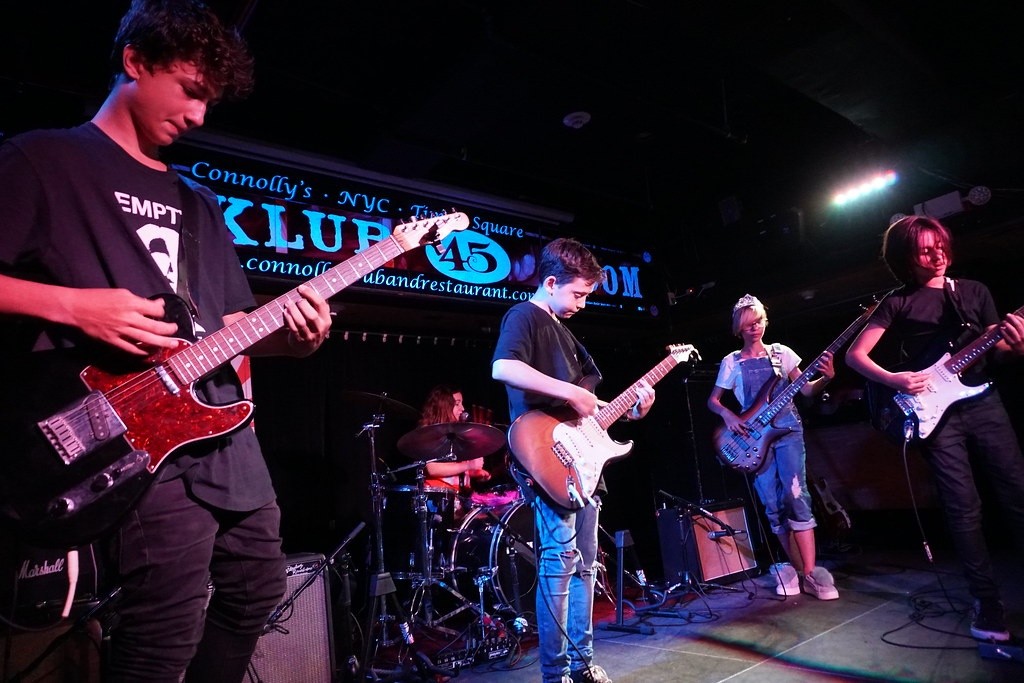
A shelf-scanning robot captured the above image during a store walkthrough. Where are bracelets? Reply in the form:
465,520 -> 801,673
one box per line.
821,377 -> 829,385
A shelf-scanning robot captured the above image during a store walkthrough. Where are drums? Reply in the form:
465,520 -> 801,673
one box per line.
449,501 -> 541,636
370,485 -> 456,581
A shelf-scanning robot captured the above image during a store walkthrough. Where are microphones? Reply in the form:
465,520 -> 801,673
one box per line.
707,530 -> 747,539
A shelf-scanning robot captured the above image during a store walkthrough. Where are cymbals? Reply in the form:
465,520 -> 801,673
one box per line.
397,422 -> 507,463
343,391 -> 422,421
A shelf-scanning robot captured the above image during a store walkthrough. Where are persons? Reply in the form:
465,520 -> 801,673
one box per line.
0,0 -> 332,682
413,388 -> 491,521
708,293 -> 839,600
843,215 -> 1024,643
492,239 -> 658,683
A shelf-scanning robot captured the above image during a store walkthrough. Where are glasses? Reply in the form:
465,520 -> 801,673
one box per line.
742,319 -> 766,331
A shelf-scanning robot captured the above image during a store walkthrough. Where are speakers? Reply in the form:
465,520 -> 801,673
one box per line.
178,553 -> 337,683
656,497 -> 763,588
8,543 -> 99,612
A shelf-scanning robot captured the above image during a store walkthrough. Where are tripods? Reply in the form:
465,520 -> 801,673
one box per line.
657,489 -> 743,609
355,400 -> 539,683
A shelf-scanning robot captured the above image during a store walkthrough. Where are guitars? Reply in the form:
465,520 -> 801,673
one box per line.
0,207 -> 477,550
710,293 -> 885,476
506,343 -> 695,514
864,306 -> 1024,451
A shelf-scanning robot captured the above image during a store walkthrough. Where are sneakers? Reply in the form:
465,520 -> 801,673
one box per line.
971,600 -> 1009,641
803,567 -> 840,600
569,664 -> 613,683
559,675 -> 574,683
776,566 -> 802,595
